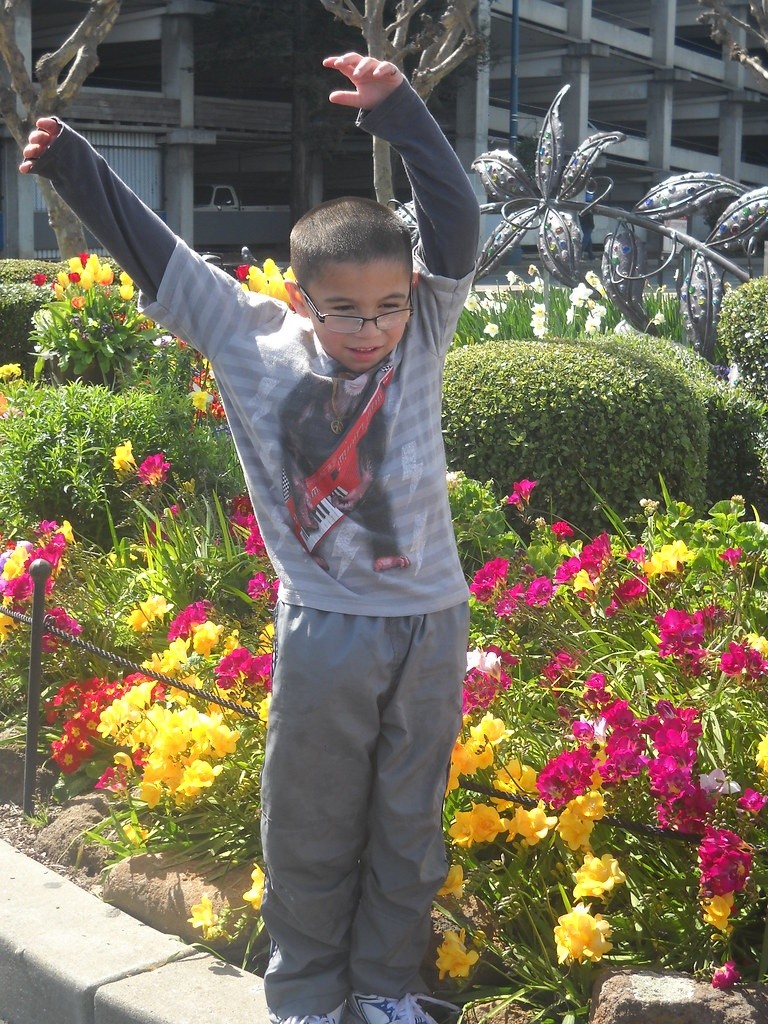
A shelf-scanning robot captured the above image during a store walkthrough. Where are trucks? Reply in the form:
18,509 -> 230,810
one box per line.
192,184 -> 291,213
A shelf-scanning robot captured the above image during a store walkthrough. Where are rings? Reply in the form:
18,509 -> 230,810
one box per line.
391,66 -> 397,76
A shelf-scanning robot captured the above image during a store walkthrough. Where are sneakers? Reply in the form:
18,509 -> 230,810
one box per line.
267,998 -> 346,1024
345,992 -> 458,1024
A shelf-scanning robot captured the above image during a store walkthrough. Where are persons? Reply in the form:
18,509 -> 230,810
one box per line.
17,52 -> 479,1024
579,196 -> 595,259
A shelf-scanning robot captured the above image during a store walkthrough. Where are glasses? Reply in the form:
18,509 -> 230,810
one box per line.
296,277 -> 415,334
